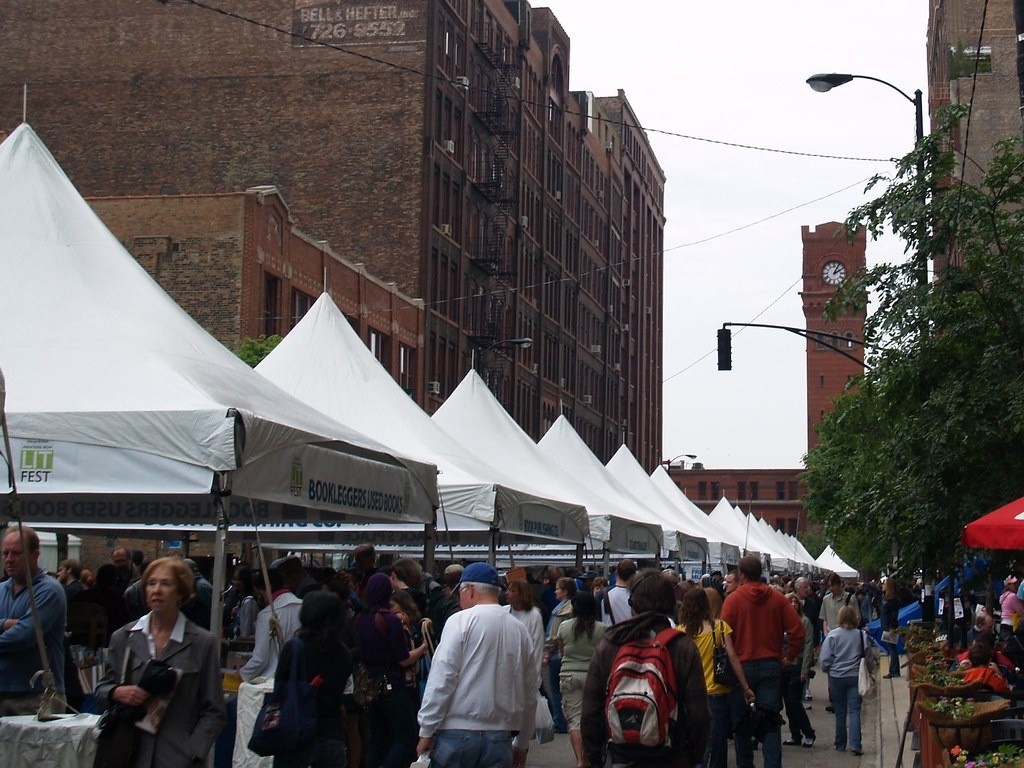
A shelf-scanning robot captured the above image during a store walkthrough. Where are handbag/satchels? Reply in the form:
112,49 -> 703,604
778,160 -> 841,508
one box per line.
93,703 -> 147,768
247,639 -> 323,758
353,665 -> 393,713
712,619 -> 737,685
536,691 -> 555,744
858,631 -> 878,698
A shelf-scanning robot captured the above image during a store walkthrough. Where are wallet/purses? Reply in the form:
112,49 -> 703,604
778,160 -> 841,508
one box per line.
130,660 -> 183,734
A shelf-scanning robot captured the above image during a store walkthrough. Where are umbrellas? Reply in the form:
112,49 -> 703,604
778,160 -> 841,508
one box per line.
961,497 -> 1024,549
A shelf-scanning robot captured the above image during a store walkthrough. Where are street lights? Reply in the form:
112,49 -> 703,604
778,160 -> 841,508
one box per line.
806,72 -> 932,348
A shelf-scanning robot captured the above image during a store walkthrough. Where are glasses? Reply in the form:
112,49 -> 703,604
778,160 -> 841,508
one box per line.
453,584 -> 473,602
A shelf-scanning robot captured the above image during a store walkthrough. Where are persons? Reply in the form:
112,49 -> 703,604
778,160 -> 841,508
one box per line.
960,574 -> 1024,696
0,528 -> 921,768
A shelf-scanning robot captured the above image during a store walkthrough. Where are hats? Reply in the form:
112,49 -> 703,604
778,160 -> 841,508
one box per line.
577,571 -> 597,579
1003,575 -> 1020,585
451,562 -> 499,594
354,546 -> 374,562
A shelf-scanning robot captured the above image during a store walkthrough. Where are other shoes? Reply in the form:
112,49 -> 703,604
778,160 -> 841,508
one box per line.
803,737 -> 815,747
783,741 -> 801,745
850,747 -> 864,755
883,674 -> 899,678
802,703 -> 812,709
825,707 -> 835,713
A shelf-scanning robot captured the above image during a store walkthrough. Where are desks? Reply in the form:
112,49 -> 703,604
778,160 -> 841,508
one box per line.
0,711 -> 103,768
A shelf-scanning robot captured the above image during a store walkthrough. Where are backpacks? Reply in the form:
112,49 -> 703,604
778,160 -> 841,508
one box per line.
604,628 -> 687,768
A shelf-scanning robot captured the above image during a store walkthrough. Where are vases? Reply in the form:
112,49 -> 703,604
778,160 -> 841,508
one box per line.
903,631 -> 1011,768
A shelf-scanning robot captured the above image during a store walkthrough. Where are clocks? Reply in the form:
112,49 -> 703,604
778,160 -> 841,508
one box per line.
821,261 -> 850,287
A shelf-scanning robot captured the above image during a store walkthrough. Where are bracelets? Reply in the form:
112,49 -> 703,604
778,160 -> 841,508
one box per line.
745,687 -> 749,691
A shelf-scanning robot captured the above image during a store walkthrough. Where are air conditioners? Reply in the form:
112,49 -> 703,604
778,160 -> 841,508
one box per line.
610,305 -> 613,312
594,239 -> 599,248
512,76 -> 520,89
442,224 -> 452,235
624,278 -> 633,287
615,362 -> 621,371
521,216 -> 531,227
456,76 -> 470,89
532,364 -> 538,372
620,419 -> 627,425
429,381 -> 440,393
623,323 -> 630,330
647,307 -> 652,313
561,378 -> 566,388
606,141 -> 617,151
597,191 -> 604,198
592,345 -> 602,355
443,140 -> 456,154
583,395 -> 592,404
556,191 -> 562,200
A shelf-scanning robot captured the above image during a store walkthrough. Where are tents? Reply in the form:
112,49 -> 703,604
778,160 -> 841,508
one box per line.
0,123 -> 860,659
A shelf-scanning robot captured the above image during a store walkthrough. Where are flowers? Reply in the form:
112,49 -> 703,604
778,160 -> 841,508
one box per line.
898,623 -> 938,641
914,672 -> 972,687
909,635 -> 948,648
925,655 -> 971,674
920,650 -> 946,666
951,743 -> 1024,768
928,695 -> 985,720
